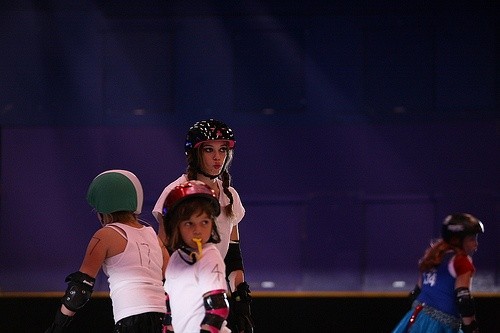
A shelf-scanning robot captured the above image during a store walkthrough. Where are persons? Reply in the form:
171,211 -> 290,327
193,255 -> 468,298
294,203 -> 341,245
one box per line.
151,117 -> 255,333
162,182 -> 230,333
393,213 -> 485,333
54,170 -> 170,333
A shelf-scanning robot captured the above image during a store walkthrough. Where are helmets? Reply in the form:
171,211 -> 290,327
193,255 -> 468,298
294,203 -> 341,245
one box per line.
440,212 -> 485,245
182,119 -> 237,152
86,169 -> 144,215
160,179 -> 221,218
